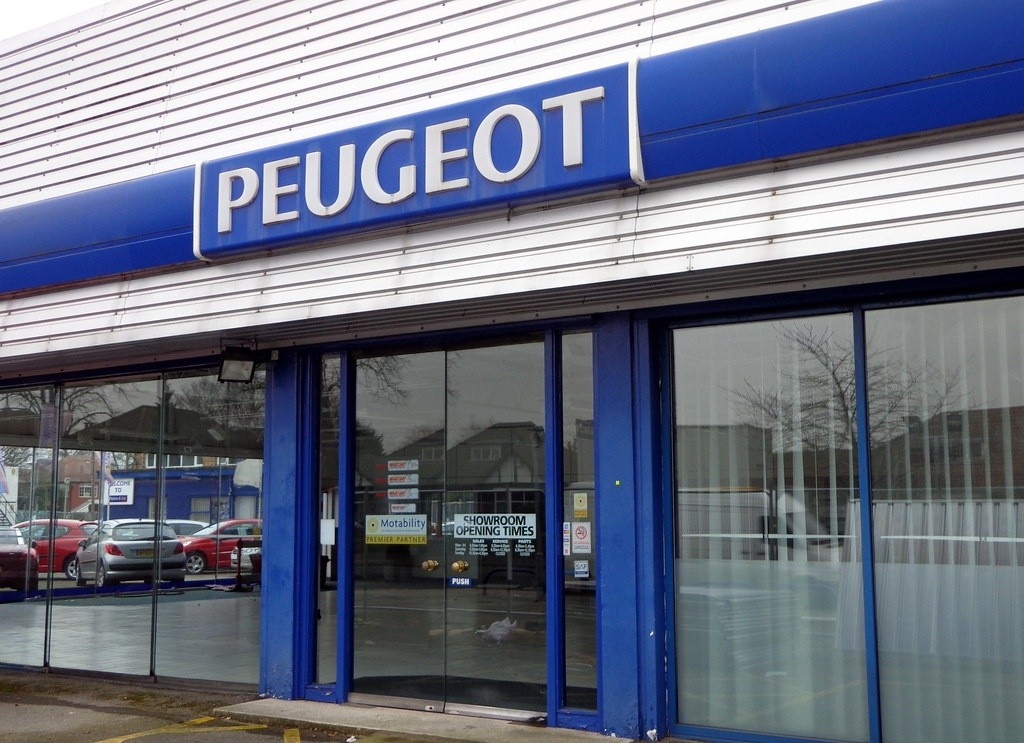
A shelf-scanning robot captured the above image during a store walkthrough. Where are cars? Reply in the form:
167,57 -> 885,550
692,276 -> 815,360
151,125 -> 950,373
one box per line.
75,517 -> 187,587
0,526 -> 39,591
178,517 -> 263,576
160,519 -> 210,539
10,518 -> 98,584
229,547 -> 261,572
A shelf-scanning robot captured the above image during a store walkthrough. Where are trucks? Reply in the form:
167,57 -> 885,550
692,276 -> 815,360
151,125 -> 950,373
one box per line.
675,482 -> 840,641
